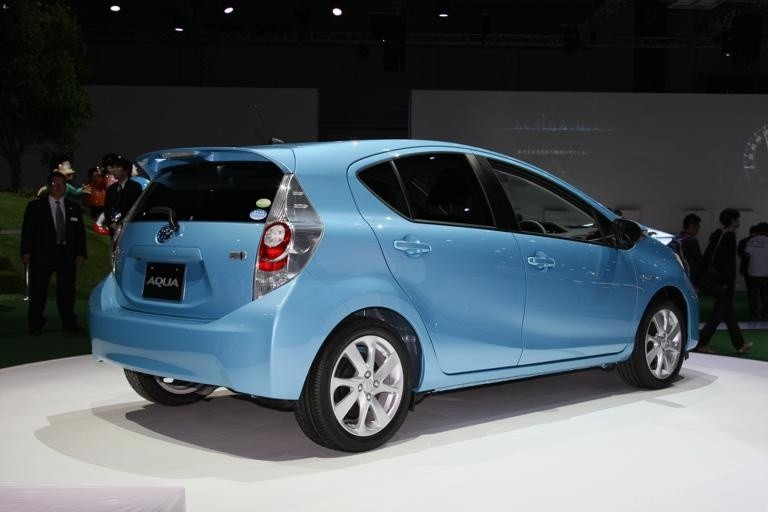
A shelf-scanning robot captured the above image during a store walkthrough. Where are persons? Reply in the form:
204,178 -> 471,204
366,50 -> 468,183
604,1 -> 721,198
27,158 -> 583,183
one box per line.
677,213 -> 701,294
694,208 -> 754,354
743,223 -> 768,321
21,153 -> 142,335
737,225 -> 757,317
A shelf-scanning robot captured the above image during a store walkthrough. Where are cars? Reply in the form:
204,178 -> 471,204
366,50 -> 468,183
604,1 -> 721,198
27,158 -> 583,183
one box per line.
87,139 -> 701,452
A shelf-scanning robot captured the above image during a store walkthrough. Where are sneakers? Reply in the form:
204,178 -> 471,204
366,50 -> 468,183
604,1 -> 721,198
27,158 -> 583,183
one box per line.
736,342 -> 753,354
697,342 -> 715,353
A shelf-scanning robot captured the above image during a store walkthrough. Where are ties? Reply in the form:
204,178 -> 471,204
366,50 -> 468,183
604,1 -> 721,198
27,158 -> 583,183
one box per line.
55,201 -> 64,243
117,184 -> 123,190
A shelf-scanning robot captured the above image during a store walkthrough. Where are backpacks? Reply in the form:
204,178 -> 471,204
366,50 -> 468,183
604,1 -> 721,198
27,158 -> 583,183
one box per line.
667,235 -> 691,277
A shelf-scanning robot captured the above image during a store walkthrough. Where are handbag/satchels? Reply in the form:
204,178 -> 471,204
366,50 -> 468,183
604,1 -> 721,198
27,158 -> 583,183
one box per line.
698,262 -> 724,297
